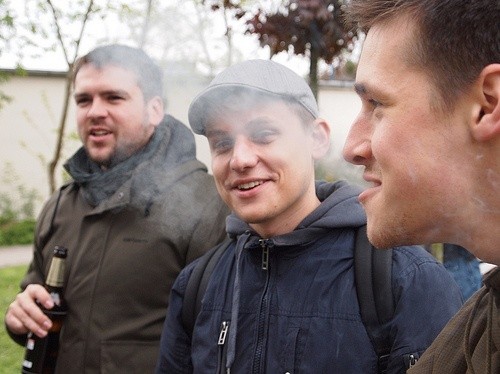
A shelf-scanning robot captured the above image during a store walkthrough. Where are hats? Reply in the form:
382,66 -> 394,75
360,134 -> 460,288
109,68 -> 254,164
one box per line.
188,59 -> 318,136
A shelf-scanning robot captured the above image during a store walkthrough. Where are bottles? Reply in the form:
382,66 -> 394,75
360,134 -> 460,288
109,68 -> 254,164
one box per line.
22,246 -> 68,374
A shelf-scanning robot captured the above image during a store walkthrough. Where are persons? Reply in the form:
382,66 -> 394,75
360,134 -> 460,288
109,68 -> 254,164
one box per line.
10,48 -> 232,374
341,0 -> 499,374
153,59 -> 465,374
